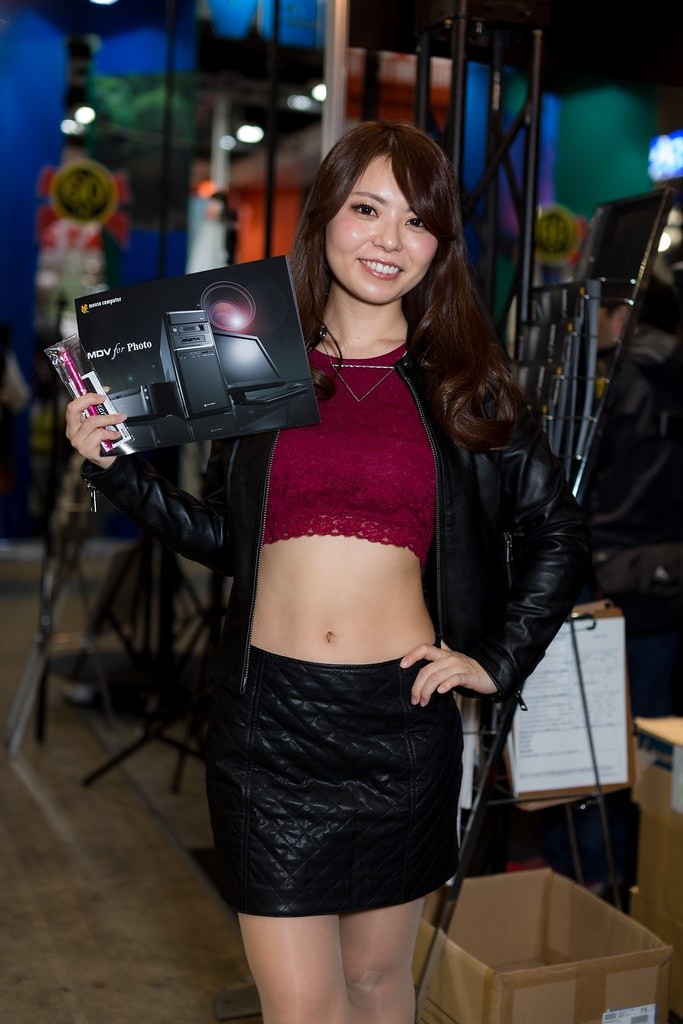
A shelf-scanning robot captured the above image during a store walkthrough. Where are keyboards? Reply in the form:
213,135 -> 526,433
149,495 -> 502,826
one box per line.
251,388 -> 308,403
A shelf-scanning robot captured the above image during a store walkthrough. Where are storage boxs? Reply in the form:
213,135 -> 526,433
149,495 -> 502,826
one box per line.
633,712 -> 683,920
629,885 -> 683,1024
413,867 -> 673,1024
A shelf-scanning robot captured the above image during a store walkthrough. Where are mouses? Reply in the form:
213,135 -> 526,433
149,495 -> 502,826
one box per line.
284,382 -> 306,388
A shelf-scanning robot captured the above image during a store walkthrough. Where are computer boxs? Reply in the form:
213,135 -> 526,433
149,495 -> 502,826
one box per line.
159,311 -> 233,421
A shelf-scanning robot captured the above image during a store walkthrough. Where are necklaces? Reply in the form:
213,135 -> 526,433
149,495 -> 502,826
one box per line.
317,337 -> 408,403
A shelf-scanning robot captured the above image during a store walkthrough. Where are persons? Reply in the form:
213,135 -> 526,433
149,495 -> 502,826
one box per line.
63,117 -> 593,1024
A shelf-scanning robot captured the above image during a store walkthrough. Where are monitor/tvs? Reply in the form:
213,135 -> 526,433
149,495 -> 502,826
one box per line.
211,329 -> 284,405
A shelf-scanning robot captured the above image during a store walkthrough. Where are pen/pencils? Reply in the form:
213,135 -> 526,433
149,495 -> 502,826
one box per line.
55,343 -> 116,450
